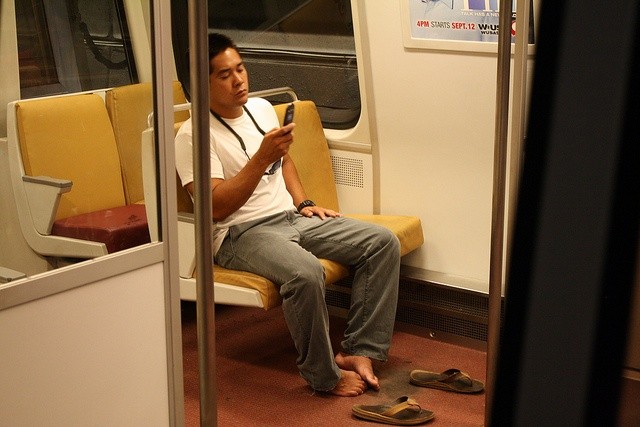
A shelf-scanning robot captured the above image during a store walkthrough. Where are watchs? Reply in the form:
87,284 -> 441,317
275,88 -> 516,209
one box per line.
297,200 -> 316,213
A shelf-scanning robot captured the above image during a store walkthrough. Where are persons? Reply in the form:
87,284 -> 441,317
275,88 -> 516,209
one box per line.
174,32 -> 401,397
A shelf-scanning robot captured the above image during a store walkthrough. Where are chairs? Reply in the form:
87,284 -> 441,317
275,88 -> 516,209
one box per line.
143,84 -> 426,301
11,79 -> 192,253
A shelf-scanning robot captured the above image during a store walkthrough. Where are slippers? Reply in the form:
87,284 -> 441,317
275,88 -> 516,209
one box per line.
410,369 -> 485,395
351,395 -> 435,427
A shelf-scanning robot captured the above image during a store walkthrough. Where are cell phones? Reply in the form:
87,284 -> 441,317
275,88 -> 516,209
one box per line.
282,103 -> 295,136
